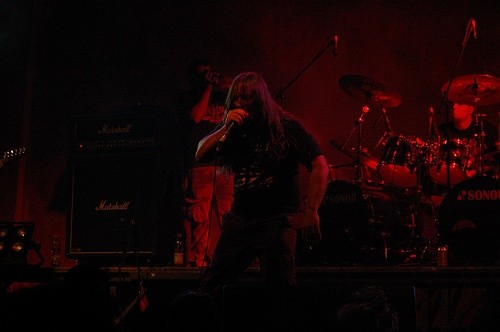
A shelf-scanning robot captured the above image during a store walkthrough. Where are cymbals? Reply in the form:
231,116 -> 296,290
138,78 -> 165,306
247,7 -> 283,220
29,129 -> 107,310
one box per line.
339,74 -> 401,108
440,74 -> 500,107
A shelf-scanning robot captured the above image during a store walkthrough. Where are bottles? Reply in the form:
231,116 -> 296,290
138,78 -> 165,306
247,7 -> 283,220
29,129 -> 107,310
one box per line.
50,232 -> 60,267
437,242 -> 447,267
173,234 -> 184,267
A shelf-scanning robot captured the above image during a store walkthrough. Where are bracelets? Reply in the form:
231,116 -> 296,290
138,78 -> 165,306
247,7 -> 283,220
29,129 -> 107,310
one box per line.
209,79 -> 216,87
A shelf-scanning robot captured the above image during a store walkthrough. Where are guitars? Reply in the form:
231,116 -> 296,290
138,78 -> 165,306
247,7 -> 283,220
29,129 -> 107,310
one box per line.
0,143 -> 34,169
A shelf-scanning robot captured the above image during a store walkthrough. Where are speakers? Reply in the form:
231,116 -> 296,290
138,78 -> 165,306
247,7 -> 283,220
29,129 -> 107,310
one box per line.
65,114 -> 190,261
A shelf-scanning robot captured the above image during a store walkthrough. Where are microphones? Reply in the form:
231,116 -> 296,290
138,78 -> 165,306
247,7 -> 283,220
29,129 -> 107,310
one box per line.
470,19 -> 476,38
218,120 -> 236,143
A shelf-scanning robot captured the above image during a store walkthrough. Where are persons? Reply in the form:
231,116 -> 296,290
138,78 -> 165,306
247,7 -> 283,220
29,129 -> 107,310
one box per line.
0,123 -> 500,332
177,59 -> 234,268
192,69 -> 329,294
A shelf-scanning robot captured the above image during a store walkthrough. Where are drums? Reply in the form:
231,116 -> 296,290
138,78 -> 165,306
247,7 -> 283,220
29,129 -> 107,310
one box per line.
432,174 -> 500,256
418,163 -> 449,208
376,134 -> 421,188
427,136 -> 477,186
316,178 -> 403,241
